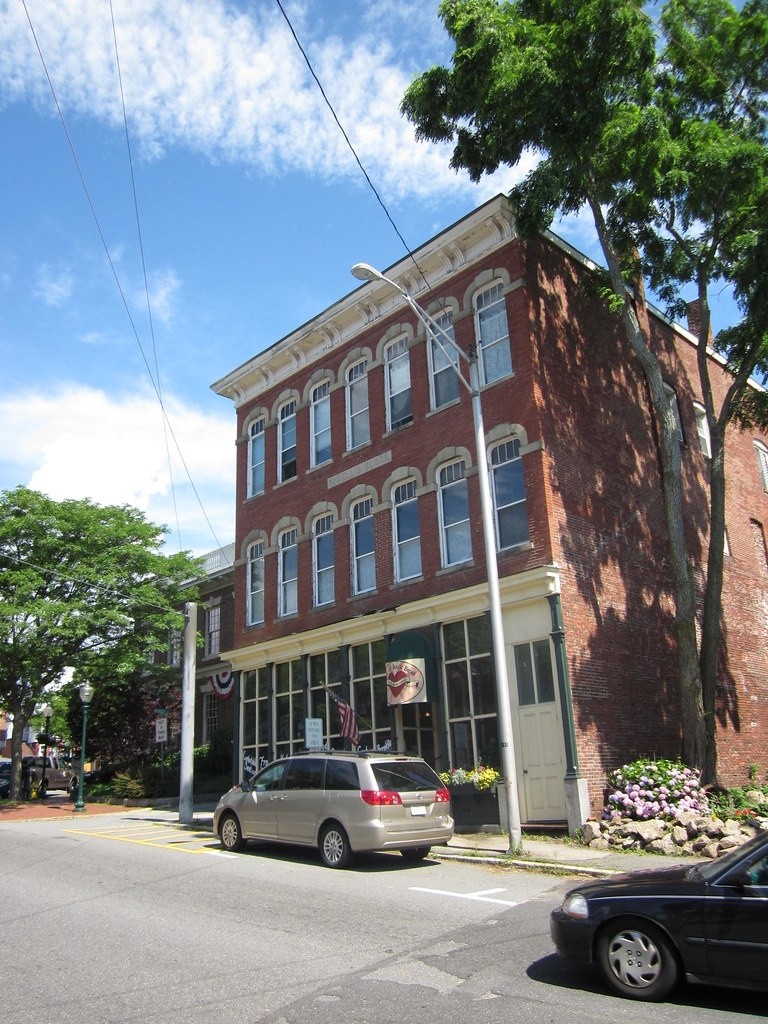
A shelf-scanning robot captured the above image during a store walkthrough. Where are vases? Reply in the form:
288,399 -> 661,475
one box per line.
447,784 -> 500,825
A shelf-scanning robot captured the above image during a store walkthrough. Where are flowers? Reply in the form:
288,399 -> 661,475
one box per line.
439,765 -> 501,791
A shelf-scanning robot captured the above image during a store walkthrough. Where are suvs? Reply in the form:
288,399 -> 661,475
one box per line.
22,755 -> 78,796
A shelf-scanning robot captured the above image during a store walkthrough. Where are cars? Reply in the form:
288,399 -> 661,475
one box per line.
0,761 -> 30,798
549,820 -> 768,1003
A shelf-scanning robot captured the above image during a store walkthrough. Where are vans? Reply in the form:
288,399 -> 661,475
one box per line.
211,749 -> 455,868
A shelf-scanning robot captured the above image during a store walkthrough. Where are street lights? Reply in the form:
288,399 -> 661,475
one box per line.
351,262 -> 525,858
75,678 -> 95,812
40,713 -> 52,798
